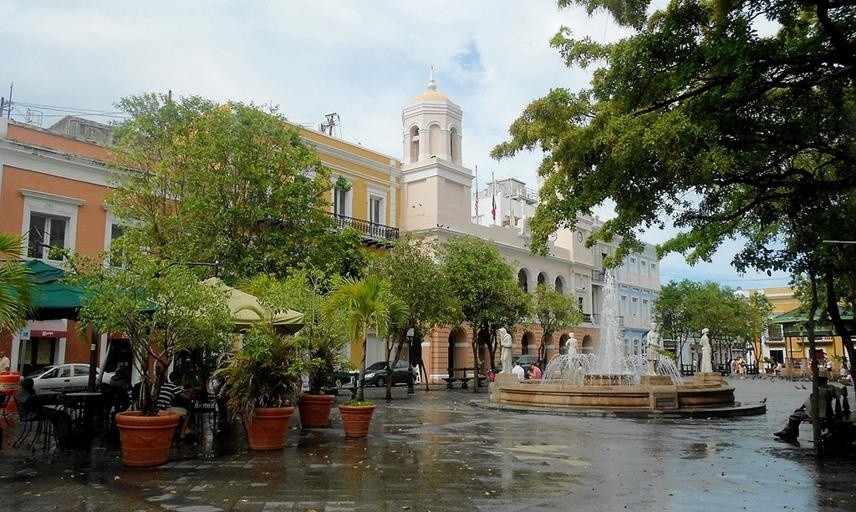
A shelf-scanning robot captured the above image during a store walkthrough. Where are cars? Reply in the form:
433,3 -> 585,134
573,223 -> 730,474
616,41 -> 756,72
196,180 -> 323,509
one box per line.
25,361 -> 117,394
358,360 -> 418,387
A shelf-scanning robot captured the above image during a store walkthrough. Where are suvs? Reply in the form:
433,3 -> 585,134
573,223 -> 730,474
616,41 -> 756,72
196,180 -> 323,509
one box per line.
496,354 -> 545,378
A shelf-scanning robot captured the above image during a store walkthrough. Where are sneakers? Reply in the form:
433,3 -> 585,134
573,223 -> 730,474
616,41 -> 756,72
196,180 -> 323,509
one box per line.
774,431 -> 797,442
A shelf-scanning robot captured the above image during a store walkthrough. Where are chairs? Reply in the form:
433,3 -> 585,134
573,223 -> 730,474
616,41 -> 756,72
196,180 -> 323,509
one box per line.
0,390 -> 58,449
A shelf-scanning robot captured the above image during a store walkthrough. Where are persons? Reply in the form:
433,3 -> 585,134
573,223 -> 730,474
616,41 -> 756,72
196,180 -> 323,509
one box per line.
512,360 -> 524,383
773,376 -> 833,442
17,378 -> 75,451
699,328 -> 714,374
212,376 -> 232,432
565,332 -> 579,357
772,361 -> 783,377
798,348 -> 832,372
0,350 -> 10,373
110,369 -> 194,438
498,329 -> 512,374
693,359 -> 697,369
527,361 -> 542,384
647,322 -> 664,376
724,356 -> 748,379
